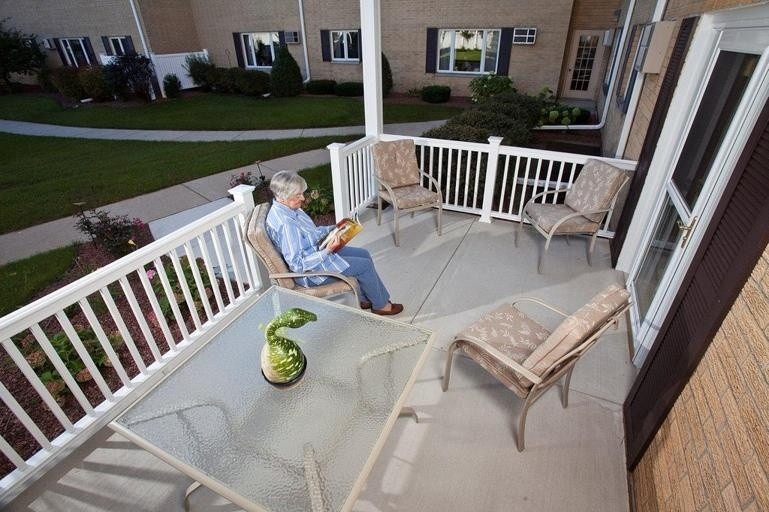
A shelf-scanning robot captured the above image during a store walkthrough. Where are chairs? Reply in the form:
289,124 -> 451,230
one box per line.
372,139 -> 443,247
441,282 -> 636,453
514,158 -> 629,274
243,202 -> 361,309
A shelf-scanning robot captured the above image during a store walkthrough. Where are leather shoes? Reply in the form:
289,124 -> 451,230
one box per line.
361,299 -> 404,315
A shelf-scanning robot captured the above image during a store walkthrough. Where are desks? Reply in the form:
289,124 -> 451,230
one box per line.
108,284 -> 438,512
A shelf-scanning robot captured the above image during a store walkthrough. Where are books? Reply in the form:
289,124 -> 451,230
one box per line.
318,207 -> 364,253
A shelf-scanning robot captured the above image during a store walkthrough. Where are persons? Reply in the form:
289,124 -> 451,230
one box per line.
266,171 -> 404,315
